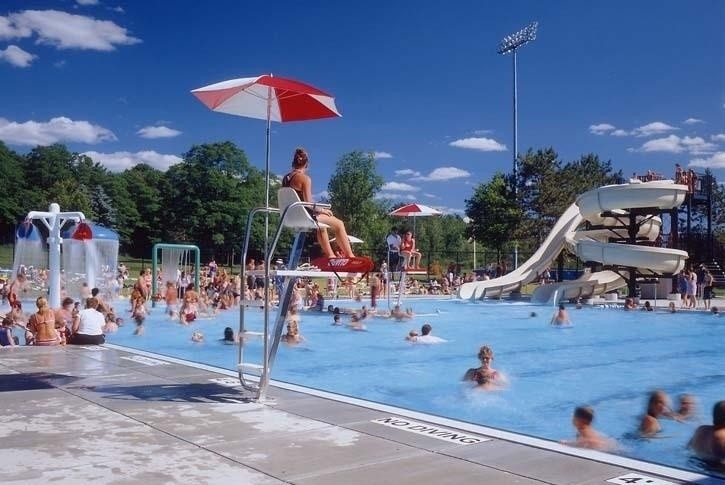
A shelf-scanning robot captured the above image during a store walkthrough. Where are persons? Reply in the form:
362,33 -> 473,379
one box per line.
560,390 -> 695,452
472,368 -> 499,391
1,262 -> 129,345
386,226 -> 403,272
400,232 -> 421,270
282,148 -> 357,260
625,267 -> 718,315
687,400 -> 725,459
128,260 -> 443,345
550,304 -> 571,327
441,261 -> 506,296
667,232 -> 673,248
544,267 -> 551,285
655,234 -> 663,247
674,163 -> 697,194
462,346 -> 505,383
632,170 -> 657,182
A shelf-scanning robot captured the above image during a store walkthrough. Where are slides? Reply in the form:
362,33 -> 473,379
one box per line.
457,179 -> 689,305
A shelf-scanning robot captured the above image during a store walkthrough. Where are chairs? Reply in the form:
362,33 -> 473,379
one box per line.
277,186 -> 333,232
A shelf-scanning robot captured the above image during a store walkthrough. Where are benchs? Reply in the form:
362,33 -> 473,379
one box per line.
388,241 -> 420,271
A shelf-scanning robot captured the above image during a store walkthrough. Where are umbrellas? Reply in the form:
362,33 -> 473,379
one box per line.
190,72 -> 343,263
388,202 -> 442,269
